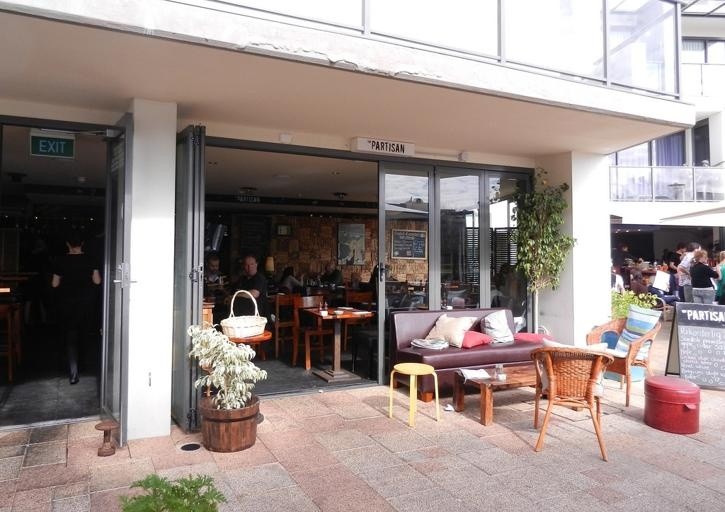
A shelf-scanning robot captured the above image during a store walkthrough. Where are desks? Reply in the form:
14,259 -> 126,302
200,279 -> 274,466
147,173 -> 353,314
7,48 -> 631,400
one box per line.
305,303 -> 375,384
200,295 -> 272,361
0,275 -> 38,385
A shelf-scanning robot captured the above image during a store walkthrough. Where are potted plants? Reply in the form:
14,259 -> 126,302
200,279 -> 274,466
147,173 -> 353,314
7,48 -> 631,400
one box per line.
184,317 -> 271,453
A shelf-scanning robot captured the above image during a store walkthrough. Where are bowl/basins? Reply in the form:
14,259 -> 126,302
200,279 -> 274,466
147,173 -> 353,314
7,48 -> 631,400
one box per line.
334,310 -> 345,315
320,311 -> 328,316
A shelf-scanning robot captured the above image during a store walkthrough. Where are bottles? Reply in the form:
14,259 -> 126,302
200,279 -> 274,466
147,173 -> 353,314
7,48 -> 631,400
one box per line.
317,300 -> 328,312
496,364 -> 504,379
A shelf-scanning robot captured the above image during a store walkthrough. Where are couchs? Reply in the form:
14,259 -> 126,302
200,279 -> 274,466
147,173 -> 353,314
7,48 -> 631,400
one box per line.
386,305 -> 560,404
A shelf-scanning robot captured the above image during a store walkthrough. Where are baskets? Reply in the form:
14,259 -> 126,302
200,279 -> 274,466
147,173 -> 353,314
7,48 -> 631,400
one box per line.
220,290 -> 268,339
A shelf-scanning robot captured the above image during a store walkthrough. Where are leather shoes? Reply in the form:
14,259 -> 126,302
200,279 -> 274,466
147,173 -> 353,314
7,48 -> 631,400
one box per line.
69,373 -> 79,384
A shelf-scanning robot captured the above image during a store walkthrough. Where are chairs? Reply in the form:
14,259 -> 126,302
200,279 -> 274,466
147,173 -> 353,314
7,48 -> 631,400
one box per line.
343,288 -> 374,353
584,315 -> 663,408
612,257 -> 725,306
262,275 -> 481,310
273,293 -> 304,359
349,306 -> 419,379
527,346 -> 617,464
291,294 -> 334,370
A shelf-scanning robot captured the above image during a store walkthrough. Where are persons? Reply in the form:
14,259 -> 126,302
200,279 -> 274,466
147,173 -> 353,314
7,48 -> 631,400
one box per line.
222,254 -> 270,329
316,261 -> 342,287
610,242 -> 725,306
277,265 -> 305,291
384,264 -> 398,281
50,231 -> 103,384
200,250 -> 232,325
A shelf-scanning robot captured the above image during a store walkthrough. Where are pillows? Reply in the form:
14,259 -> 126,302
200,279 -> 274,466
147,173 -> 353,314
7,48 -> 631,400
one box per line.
462,330 -> 493,350
513,331 -> 554,345
616,304 -> 663,360
424,312 -> 481,350
478,309 -> 515,347
538,336 -> 610,399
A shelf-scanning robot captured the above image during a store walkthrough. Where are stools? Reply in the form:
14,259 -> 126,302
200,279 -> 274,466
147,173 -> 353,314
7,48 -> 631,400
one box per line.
387,361 -> 442,428
642,374 -> 702,435
